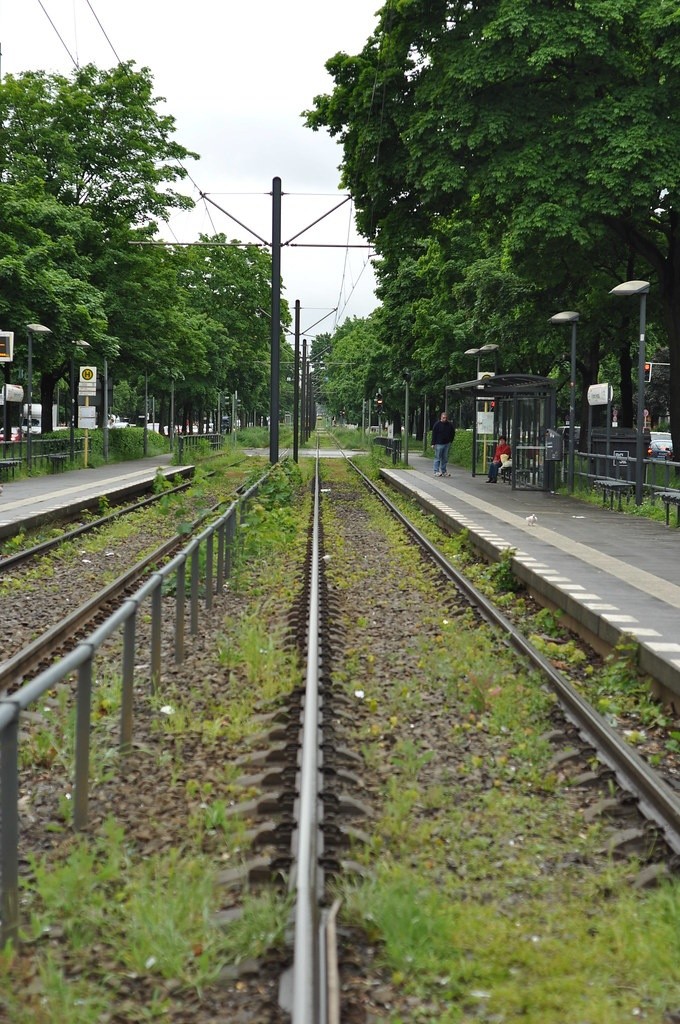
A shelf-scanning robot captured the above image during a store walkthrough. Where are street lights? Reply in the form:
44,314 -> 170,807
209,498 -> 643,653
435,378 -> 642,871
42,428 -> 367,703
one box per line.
547,310 -> 579,496
609,280 -> 650,507
480,344 -> 500,469
464,348 -> 480,467
27,323 -> 53,474
69,339 -> 91,461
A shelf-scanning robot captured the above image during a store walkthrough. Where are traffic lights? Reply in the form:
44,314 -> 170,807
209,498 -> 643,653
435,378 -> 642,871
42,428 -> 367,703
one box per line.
645,362 -> 652,382
378,394 -> 383,404
376,404 -> 383,415
490,401 -> 495,412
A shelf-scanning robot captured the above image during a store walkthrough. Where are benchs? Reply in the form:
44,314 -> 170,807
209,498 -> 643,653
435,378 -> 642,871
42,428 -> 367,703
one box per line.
498,467 -> 512,484
50,455 -> 68,474
594,480 -> 632,512
515,468 -> 531,488
654,492 -> 680,526
0,460 -> 22,481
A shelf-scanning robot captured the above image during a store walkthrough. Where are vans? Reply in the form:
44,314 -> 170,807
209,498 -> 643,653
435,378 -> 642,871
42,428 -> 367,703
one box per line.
556,426 -> 581,451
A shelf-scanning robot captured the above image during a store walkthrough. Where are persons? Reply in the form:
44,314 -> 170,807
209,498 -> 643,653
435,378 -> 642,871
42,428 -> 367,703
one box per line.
486,436 -> 511,483
431,412 -> 455,476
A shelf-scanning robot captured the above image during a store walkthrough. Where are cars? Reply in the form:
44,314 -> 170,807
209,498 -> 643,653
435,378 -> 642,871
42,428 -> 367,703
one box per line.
650,431 -> 672,440
648,440 -> 674,461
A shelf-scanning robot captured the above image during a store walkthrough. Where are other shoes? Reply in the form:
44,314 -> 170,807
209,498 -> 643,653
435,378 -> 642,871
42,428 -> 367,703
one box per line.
486,478 -> 491,483
442,473 -> 450,477
434,472 -> 442,477
490,479 -> 497,483
